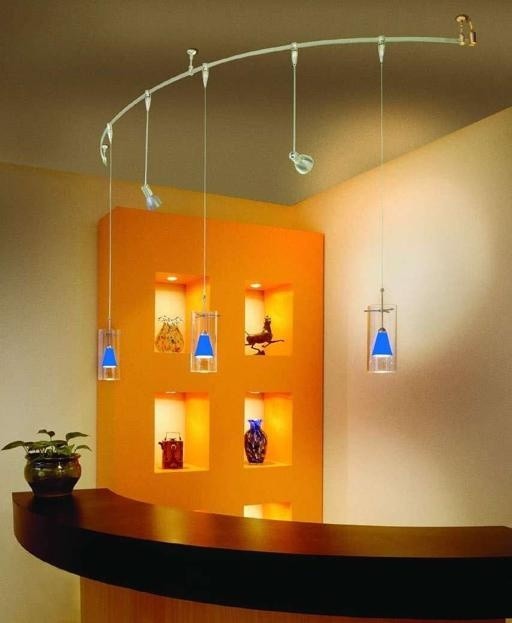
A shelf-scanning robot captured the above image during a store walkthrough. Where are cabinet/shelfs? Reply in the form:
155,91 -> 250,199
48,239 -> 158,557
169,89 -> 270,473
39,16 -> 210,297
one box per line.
95,207 -> 324,522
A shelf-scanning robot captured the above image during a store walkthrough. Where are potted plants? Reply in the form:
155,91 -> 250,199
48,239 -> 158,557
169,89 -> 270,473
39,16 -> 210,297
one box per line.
0,424 -> 93,499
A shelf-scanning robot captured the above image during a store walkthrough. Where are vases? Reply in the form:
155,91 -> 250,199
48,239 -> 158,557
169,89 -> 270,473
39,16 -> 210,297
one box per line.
244,418 -> 267,463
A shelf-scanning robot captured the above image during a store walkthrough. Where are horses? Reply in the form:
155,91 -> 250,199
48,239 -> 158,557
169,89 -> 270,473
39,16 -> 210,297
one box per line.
245,315 -> 285,352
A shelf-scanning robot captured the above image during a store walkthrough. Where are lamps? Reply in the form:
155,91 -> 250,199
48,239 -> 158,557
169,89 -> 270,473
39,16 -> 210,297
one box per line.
288,47 -> 314,175
137,93 -> 162,211
96,125 -> 121,385
366,45 -> 397,375
191,66 -> 219,373
